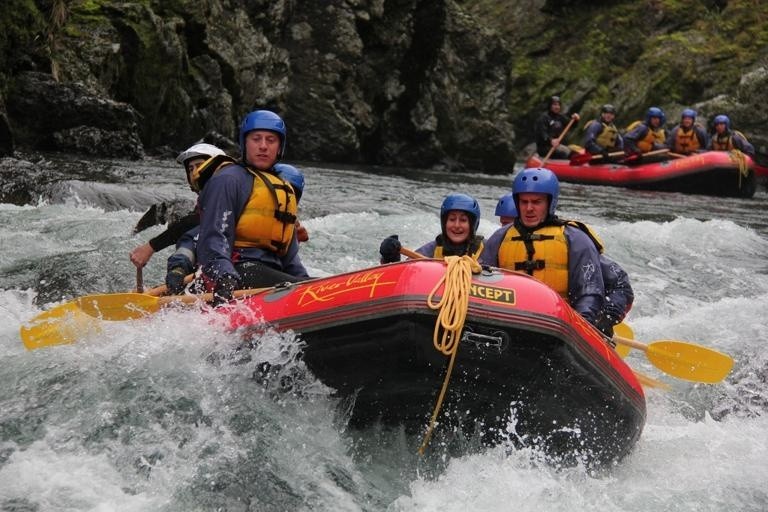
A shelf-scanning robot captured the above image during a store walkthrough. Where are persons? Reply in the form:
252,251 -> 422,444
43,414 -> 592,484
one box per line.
274,165 -> 305,207
536,92 -> 757,160
374,193 -> 488,264
494,189 -> 633,339
480,166 -> 606,325
191,108 -> 318,297
125,131 -> 236,294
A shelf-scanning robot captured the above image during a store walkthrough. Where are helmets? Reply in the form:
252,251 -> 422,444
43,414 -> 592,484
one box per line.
494,192 -> 520,218
439,191 -> 481,240
548,94 -> 562,112
511,167 -> 558,217
601,104 -> 617,115
679,109 -> 697,128
644,106 -> 665,129
175,143 -> 226,166
238,108 -> 286,161
540,198 -> 546,199
713,113 -> 731,133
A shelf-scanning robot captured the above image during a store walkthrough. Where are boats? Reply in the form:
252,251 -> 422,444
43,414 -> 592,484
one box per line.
195,255 -> 654,473
519,146 -> 762,205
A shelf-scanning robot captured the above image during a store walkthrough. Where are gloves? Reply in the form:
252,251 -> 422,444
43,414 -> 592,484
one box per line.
164,268 -> 185,294
379,234 -> 402,263
211,277 -> 238,308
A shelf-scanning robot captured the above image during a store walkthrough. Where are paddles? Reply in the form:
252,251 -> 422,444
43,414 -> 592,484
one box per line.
80,286 -> 276,320
614,334 -> 734,384
569,151 -> 625,166
20,272 -> 195,350
400,245 -> 634,357
616,148 -> 671,164
